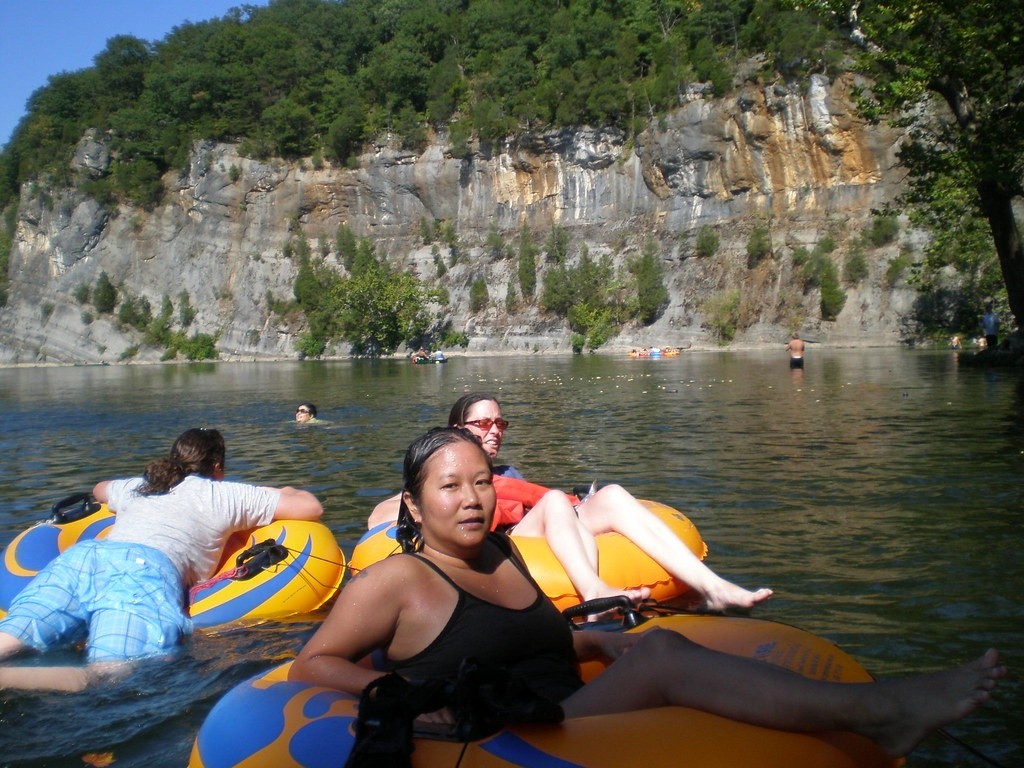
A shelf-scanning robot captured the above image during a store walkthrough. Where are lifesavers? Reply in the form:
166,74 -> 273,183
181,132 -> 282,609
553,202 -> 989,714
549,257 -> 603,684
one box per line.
187,596 -> 903,768
0,495 -> 343,631
349,498 -> 710,620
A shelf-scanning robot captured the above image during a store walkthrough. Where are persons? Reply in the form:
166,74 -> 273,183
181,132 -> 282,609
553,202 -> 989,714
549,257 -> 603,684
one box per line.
948,296 -> 1003,349
418,346 -> 446,360
288,424 -> 1007,756
367,391 -> 772,624
0,428 -> 324,693
295,403 -> 332,426
786,332 -> 805,371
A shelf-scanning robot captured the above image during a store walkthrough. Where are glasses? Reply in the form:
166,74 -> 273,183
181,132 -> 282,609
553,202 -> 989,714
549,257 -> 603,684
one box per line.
296,408 -> 311,414
465,417 -> 509,430
